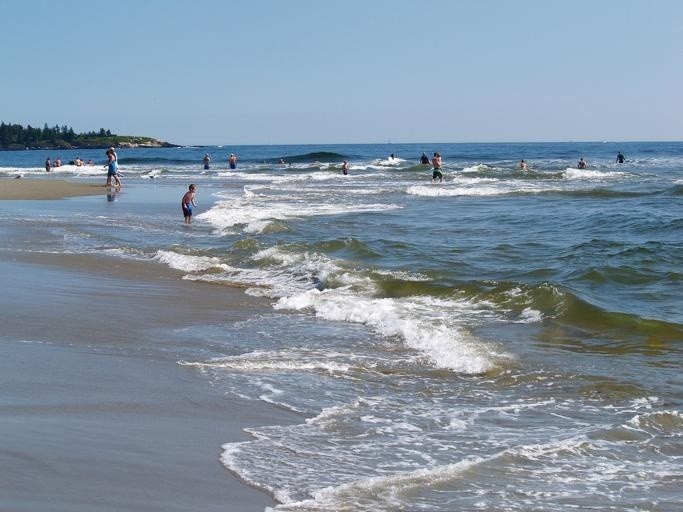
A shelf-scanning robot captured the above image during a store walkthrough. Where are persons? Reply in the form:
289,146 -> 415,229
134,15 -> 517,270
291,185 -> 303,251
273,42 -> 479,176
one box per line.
436,153 -> 441,163
391,153 -> 394,160
45,157 -> 93,172
181,183 -> 198,223
432,152 -> 443,182
228,153 -> 236,169
615,151 -> 625,163
203,153 -> 210,169
342,160 -> 349,175
278,159 -> 284,164
578,158 -> 586,169
520,159 -> 528,169
103,146 -> 121,186
420,152 -> 430,164
106,187 -> 120,202
